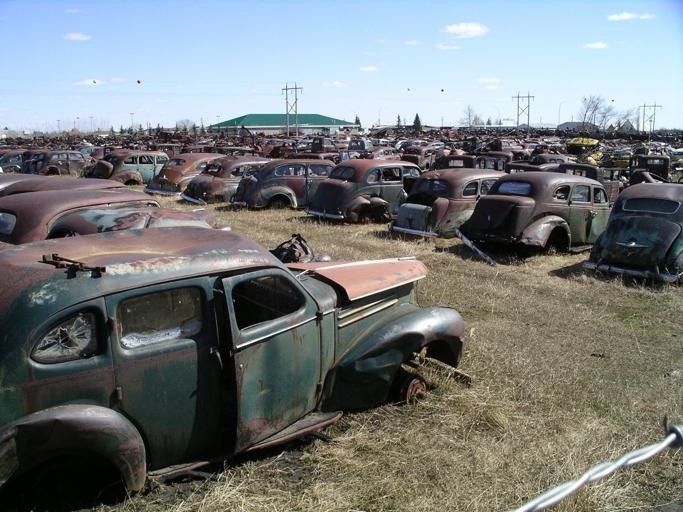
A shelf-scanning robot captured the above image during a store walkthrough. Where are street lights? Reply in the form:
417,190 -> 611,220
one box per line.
649,119 -> 652,142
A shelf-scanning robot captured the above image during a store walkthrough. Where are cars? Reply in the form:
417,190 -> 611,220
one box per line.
470,172 -> 614,255
0,229 -> 473,511
583,182 -> 683,283
0,191 -> 161,246
304,158 -> 423,225
388,168 -> 510,237
0,130 -> 683,186
182,156 -> 271,206
46,207 -> 228,239
234,159 -> 338,211
1,178 -> 127,199
143,153 -> 228,197
